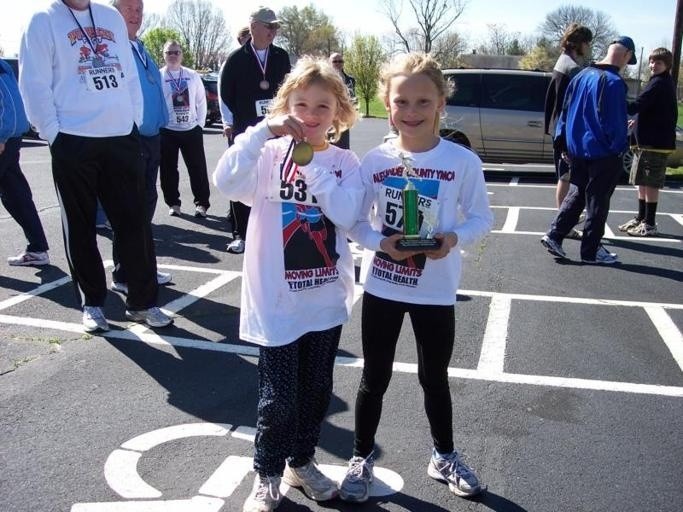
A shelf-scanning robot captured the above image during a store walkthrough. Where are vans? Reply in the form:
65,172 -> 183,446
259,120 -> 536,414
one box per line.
436,67 -> 683,177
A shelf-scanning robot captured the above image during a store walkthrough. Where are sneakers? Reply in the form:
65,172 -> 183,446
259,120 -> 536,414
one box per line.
427,448 -> 480,496
541,214 -> 657,263
169,205 -> 180,216
195,206 -> 206,216
83,270 -> 174,332
8,251 -> 50,266
227,240 -> 245,253
243,456 -> 372,512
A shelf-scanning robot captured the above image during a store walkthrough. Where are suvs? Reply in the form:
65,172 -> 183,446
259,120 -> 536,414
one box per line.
194,69 -> 222,126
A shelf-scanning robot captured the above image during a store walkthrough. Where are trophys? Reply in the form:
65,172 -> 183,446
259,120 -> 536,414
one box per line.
395,152 -> 441,249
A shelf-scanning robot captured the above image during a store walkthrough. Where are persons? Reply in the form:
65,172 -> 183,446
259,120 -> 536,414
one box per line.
329,50 -> 356,152
91,0 -> 175,246
339,51 -> 492,502
211,55 -> 359,512
220,7 -> 292,258
157,38 -> 210,223
539,32 -> 637,263
541,24 -> 607,242
17,0 -> 176,331
0,59 -> 52,270
618,46 -> 679,236
215,26 -> 253,149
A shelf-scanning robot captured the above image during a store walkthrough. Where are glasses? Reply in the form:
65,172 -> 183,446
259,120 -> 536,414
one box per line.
165,51 -> 181,55
264,24 -> 280,29
332,60 -> 343,63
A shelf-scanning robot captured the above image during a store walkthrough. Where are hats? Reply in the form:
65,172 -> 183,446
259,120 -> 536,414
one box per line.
610,36 -> 637,64
250,8 -> 283,23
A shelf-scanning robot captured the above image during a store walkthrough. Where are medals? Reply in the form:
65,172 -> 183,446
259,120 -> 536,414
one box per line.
147,71 -> 155,83
258,79 -> 270,90
91,57 -> 102,67
288,139 -> 315,168
175,94 -> 184,102
